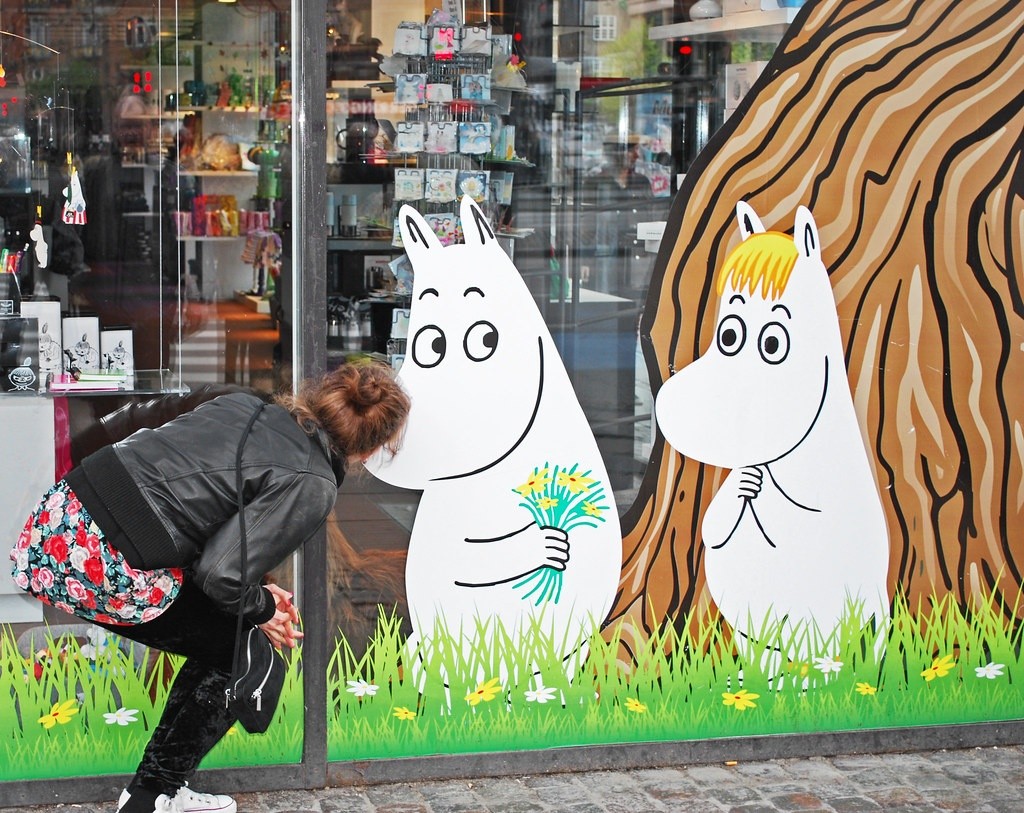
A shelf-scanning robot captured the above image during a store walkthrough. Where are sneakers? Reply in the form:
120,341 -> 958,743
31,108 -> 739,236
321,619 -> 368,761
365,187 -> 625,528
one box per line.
114,786 -> 237,813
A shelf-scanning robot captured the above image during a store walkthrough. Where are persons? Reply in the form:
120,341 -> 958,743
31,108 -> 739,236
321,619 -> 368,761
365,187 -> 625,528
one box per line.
68,95 -> 195,418
10,359 -> 412,813
51,136 -> 94,281
581,128 -> 654,405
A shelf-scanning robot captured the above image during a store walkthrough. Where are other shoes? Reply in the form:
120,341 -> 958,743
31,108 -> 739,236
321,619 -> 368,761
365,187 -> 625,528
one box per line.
71,263 -> 91,272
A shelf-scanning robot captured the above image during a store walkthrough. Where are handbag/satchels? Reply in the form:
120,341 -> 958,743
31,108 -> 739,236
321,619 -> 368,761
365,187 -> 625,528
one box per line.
225,626 -> 285,735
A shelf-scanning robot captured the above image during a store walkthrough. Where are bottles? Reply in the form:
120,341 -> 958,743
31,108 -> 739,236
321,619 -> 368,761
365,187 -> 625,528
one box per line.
215,64 -> 276,110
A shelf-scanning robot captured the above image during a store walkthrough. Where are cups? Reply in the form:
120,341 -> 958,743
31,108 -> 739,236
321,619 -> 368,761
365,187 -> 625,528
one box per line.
325,191 -> 358,238
336,119 -> 374,163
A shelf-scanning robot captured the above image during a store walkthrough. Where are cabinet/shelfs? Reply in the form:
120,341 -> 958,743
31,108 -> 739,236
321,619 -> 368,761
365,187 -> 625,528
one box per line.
121,39 -> 281,243
320,84 -> 534,359
